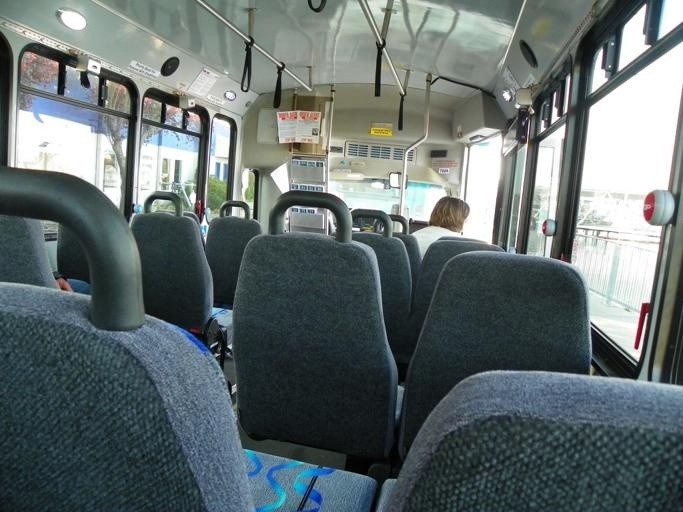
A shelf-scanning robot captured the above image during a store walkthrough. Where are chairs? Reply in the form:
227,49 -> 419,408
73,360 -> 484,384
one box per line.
376,367 -> 682,512
0,165 -> 377,511
154,209 -> 205,250
229,190 -> 403,462
394,249 -> 591,465
205,200 -> 262,311
128,190 -> 233,353
55,218 -> 95,290
341,208 -> 508,360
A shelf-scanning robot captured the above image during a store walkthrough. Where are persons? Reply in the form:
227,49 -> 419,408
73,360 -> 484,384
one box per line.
410,196 -> 470,261
